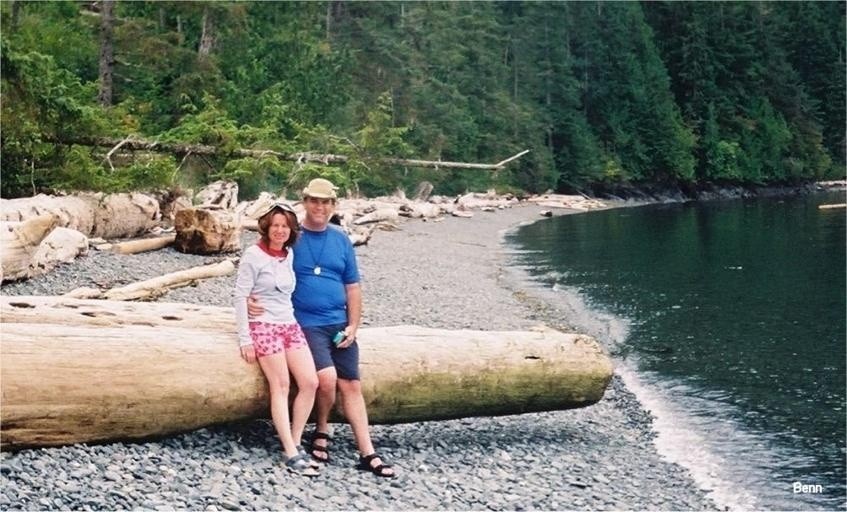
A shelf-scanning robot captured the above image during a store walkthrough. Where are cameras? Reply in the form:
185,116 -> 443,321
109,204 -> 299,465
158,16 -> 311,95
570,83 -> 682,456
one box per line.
332,332 -> 344,345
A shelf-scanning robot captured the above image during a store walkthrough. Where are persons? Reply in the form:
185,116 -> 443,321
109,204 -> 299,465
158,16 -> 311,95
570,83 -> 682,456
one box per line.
246,176 -> 396,479
235,202 -> 321,480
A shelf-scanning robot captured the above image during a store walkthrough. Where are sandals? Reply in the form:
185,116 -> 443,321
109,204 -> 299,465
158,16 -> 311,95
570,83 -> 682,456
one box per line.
312,429 -> 329,462
359,453 -> 394,476
281,445 -> 320,476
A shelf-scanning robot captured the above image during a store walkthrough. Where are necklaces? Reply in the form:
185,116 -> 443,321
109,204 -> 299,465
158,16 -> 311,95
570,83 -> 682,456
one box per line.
266,244 -> 297,296
300,221 -> 327,276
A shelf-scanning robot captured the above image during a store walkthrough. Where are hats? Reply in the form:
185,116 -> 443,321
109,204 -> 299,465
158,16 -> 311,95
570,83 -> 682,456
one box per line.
251,202 -> 297,220
302,178 -> 340,199
